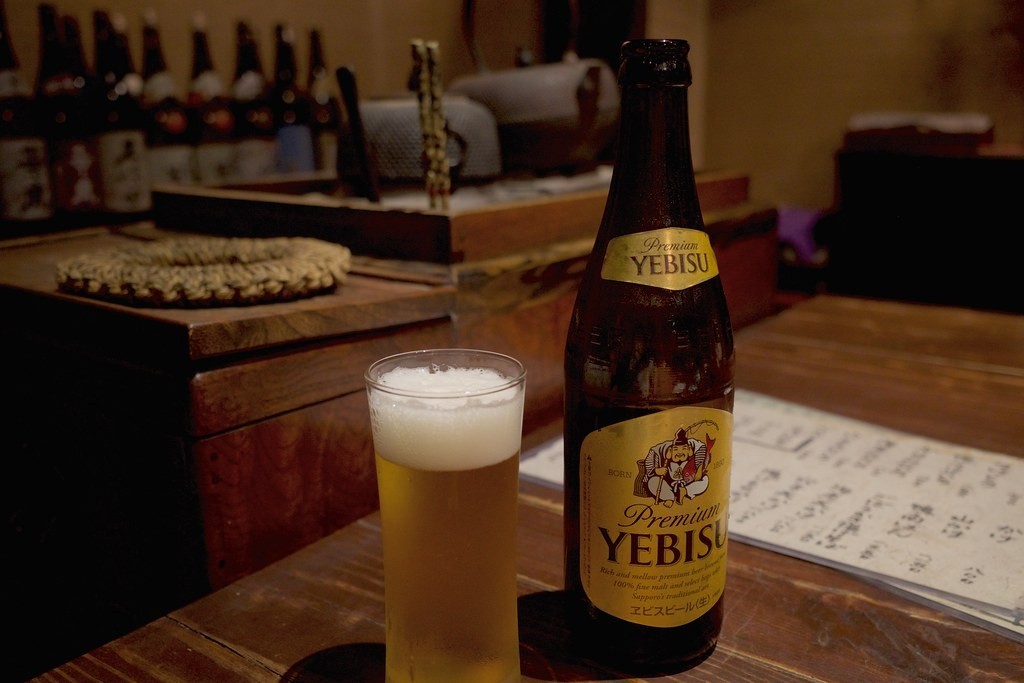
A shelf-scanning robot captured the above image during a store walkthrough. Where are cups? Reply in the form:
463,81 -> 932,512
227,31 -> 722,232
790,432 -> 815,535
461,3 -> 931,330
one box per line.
364,348 -> 527,683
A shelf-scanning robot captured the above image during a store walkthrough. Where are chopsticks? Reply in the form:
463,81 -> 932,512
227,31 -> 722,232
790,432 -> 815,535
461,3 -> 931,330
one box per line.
411,40 -> 451,209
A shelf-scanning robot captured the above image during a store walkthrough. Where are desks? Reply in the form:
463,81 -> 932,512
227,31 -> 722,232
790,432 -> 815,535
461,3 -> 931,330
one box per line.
0,206 -> 781,683
24,295 -> 1024,682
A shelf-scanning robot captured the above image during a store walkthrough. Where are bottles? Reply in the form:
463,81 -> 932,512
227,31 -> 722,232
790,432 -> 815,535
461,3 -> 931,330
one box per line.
189,10 -> 236,186
565,38 -> 736,675
274,22 -> 315,173
0,1 -> 54,236
307,26 -> 345,172
143,10 -> 194,184
100,12 -> 153,226
36,2 -> 102,228
233,21 -> 278,178
